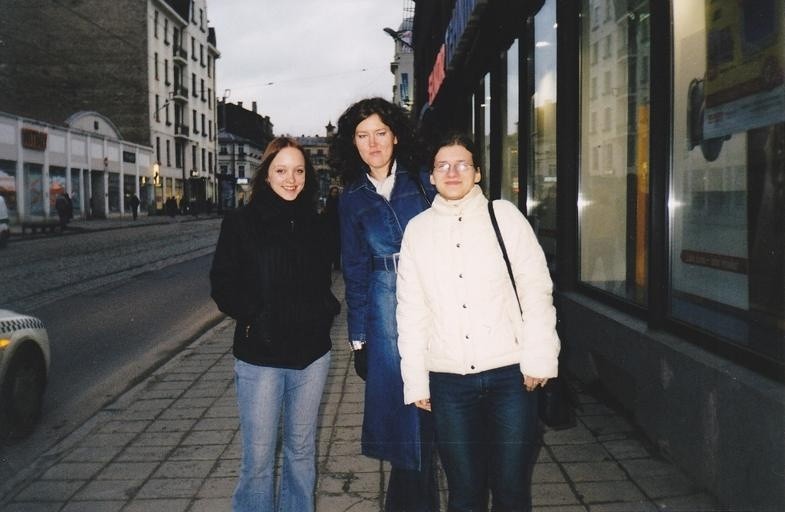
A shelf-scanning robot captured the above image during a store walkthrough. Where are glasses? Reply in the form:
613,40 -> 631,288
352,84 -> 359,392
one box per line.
434,161 -> 472,174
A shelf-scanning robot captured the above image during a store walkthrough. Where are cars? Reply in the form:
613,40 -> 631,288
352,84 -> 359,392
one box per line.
1,308 -> 52,441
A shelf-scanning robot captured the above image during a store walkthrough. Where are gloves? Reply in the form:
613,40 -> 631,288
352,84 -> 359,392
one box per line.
349,341 -> 369,381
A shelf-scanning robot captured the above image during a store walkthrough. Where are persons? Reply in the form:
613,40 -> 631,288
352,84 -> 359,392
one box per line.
209,136 -> 341,512
0,192 -> 187,244
326,186 -> 341,271
327,97 -> 439,512
396,134 -> 561,512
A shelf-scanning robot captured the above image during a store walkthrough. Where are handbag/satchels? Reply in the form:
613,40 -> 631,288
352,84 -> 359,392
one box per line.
537,376 -> 576,431
